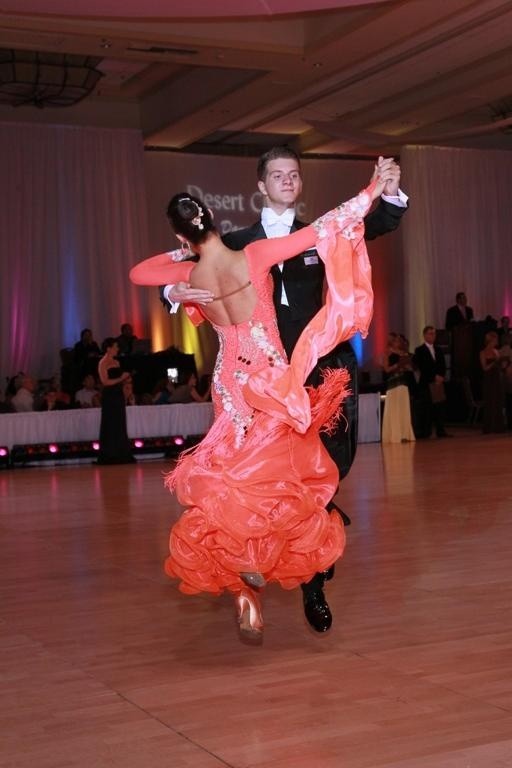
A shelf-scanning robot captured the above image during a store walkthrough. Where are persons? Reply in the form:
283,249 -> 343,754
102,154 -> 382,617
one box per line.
381,291 -> 511,443
129,152 -> 396,642
1,322 -> 217,467
159,148 -> 408,632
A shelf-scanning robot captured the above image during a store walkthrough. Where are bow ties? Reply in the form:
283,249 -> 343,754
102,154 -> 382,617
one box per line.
261,207 -> 295,226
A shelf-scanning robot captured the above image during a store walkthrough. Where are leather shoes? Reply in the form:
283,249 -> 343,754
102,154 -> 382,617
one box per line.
303,589 -> 332,632
325,501 -> 351,525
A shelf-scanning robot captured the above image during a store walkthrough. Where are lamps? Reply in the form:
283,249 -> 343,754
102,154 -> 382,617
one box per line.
1,49 -> 107,111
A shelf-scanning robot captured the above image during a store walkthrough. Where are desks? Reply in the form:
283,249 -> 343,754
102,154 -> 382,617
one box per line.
1,393 -> 383,450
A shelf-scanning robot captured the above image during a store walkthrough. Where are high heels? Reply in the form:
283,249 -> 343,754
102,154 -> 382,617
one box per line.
236,588 -> 264,639
